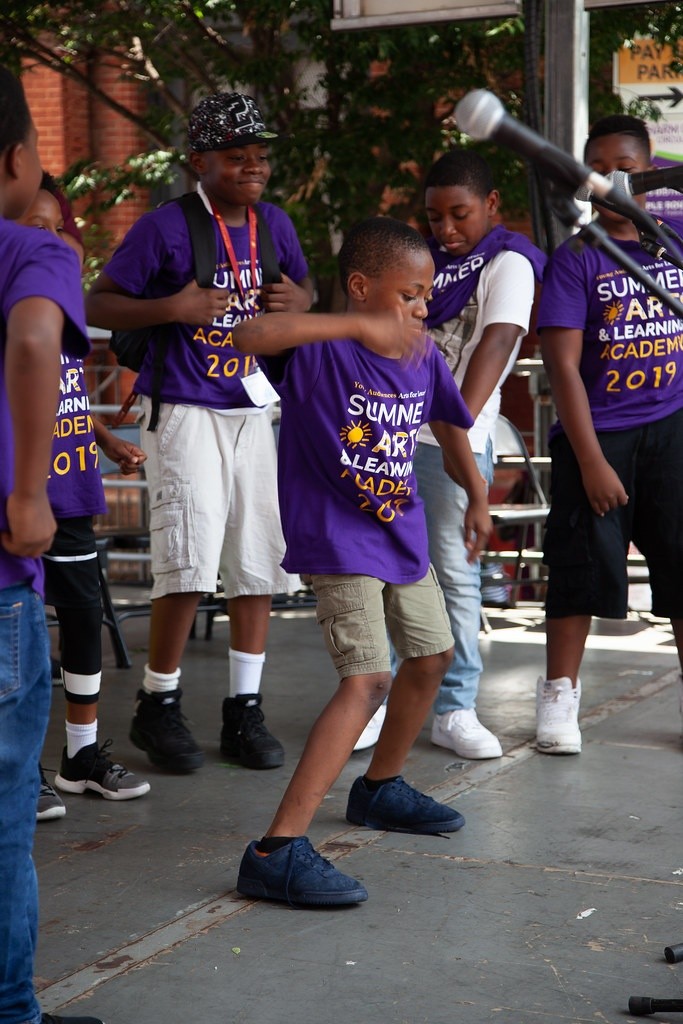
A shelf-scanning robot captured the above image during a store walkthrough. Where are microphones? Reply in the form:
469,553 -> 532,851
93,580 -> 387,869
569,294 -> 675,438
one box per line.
455,88 -> 683,242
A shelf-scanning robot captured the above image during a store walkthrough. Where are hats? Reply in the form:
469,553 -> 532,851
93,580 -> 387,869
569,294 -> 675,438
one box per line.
190,92 -> 277,151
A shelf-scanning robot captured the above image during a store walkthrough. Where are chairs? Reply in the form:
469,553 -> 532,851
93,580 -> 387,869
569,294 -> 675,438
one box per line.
51,410 -> 554,668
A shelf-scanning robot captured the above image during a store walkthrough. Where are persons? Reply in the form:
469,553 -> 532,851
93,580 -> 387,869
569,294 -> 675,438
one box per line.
0,63 -> 102,1024
83,92 -> 314,772
13,171 -> 151,820
233,217 -> 493,908
353,150 -> 545,758
536,115 -> 683,753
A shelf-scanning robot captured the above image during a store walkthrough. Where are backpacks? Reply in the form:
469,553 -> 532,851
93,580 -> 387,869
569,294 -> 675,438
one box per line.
109,193 -> 283,431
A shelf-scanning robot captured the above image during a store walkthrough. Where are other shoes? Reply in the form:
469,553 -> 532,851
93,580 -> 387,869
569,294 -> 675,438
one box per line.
49,657 -> 62,685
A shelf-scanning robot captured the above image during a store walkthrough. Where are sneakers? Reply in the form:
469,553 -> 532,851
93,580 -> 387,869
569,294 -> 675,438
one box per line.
431,708 -> 502,759
536,676 -> 582,753
36,764 -> 66,820
346,776 -> 465,832
55,739 -> 152,801
220,693 -> 284,769
353,704 -> 387,750
236,836 -> 368,909
129,688 -> 204,772
